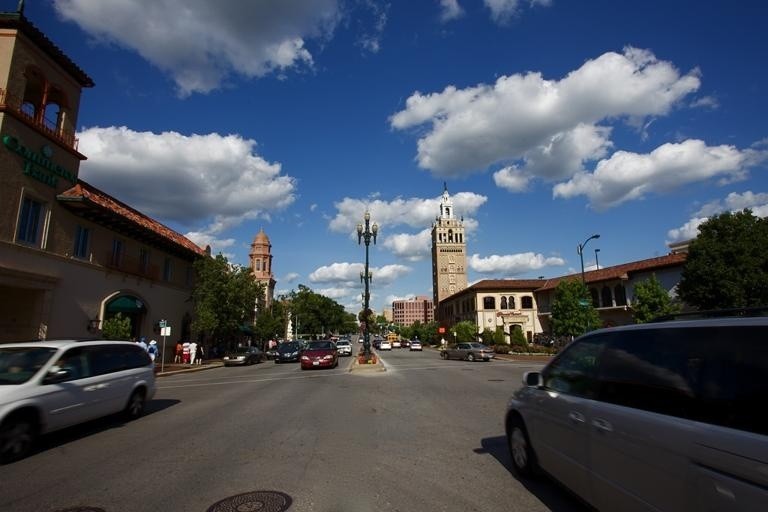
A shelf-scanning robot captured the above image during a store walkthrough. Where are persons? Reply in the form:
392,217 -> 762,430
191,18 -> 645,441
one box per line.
174,340 -> 182,363
135,336 -> 147,349
271,337 -> 277,349
147,339 -> 159,365
195,342 -> 204,364
189,342 -> 197,364
181,339 -> 191,364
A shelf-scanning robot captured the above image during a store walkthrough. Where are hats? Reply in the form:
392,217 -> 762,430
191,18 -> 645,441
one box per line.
148,340 -> 157,345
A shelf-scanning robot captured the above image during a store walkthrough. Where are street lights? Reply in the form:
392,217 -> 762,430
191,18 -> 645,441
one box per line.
356,211 -> 380,365
577,232 -> 600,291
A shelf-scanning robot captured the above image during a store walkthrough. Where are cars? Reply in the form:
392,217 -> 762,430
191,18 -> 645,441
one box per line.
329,333 -> 363,358
369,331 -> 423,352
440,341 -> 495,362
274,341 -> 304,363
301,340 -> 338,370
266,347 -> 279,361
222,346 -> 264,367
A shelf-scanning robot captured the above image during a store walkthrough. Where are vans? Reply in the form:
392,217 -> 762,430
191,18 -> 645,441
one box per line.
506,317 -> 767,512
0,337 -> 157,462
